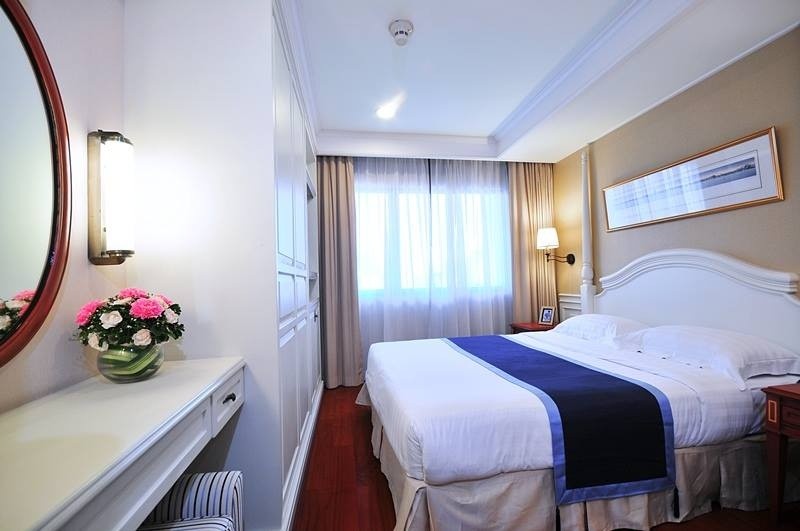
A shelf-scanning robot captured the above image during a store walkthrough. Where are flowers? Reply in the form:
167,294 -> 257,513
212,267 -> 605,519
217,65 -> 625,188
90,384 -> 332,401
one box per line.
68,288 -> 183,352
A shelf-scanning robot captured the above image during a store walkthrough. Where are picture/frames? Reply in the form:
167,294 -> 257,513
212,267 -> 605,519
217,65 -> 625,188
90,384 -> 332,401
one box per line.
538,306 -> 555,326
600,126 -> 785,234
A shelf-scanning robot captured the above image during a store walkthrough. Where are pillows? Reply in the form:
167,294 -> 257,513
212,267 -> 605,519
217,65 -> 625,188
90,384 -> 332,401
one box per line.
627,321 -> 797,388
550,314 -> 649,348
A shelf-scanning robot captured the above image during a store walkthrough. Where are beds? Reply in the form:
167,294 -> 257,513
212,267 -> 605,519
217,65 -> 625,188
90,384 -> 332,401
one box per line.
356,250 -> 800,531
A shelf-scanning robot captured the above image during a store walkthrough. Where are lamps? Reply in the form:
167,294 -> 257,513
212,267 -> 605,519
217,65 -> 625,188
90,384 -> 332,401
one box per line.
86,127 -> 136,267
536,226 -> 575,264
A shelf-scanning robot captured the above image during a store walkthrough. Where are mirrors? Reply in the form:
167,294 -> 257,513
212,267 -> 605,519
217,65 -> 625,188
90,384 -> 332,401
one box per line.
0,0 -> 72,369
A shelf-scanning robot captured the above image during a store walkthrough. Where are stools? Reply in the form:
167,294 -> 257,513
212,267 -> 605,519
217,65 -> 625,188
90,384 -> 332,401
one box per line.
137,471 -> 243,531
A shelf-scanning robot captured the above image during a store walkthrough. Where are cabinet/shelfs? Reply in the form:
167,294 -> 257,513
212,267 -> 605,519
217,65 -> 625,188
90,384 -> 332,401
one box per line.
0,355 -> 246,531
125,2 -> 321,531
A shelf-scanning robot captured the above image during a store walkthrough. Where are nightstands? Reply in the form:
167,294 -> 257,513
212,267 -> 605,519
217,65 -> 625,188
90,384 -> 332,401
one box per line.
509,323 -> 555,334
761,385 -> 800,531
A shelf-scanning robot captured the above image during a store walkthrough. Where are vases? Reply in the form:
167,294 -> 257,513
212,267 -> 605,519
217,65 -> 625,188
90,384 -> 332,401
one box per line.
97,346 -> 165,382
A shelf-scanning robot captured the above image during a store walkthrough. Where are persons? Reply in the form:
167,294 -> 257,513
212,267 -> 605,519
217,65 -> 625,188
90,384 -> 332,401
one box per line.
544,311 -> 551,320
